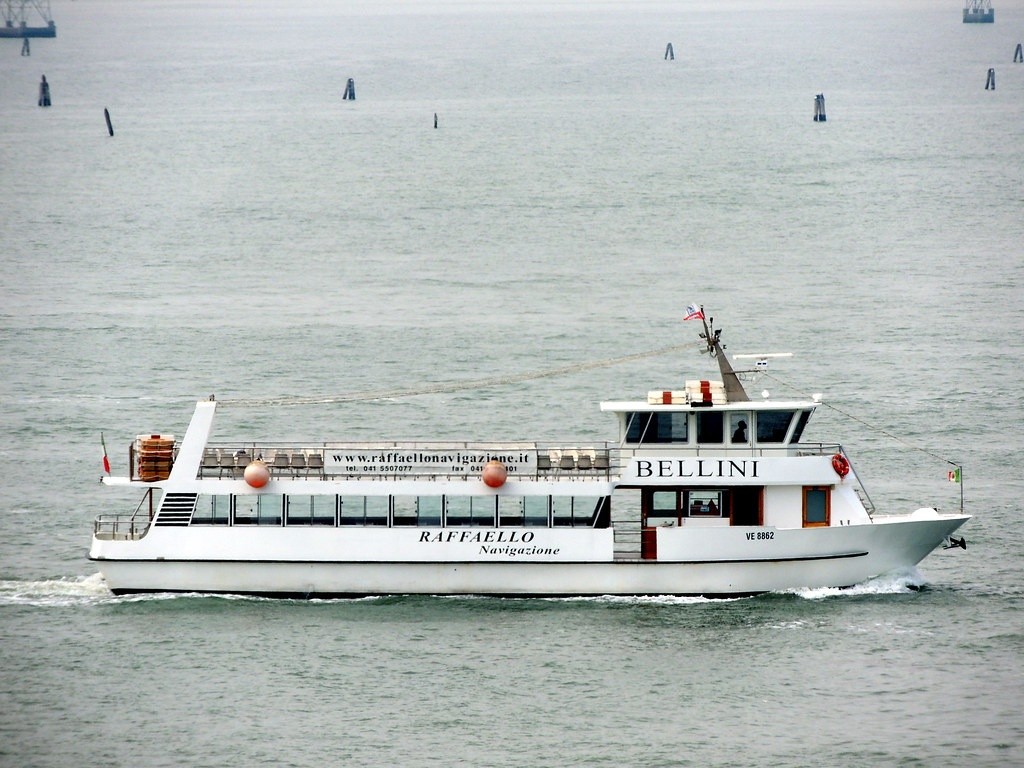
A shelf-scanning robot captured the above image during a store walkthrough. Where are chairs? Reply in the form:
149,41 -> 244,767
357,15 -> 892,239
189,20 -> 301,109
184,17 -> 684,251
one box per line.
201,454 -> 325,481
537,454 -> 609,482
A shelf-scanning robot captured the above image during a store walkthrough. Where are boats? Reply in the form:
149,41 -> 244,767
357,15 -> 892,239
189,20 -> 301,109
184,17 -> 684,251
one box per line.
85,302 -> 974,601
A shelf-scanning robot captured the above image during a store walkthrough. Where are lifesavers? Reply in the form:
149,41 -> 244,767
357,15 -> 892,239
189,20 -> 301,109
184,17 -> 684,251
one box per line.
832,454 -> 849,475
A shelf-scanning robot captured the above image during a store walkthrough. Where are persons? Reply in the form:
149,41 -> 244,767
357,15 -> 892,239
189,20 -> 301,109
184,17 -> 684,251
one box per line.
731,420 -> 748,443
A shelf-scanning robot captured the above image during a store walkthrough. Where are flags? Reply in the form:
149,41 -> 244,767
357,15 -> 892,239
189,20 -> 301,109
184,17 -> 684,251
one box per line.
682,302 -> 705,320
948,468 -> 960,483
